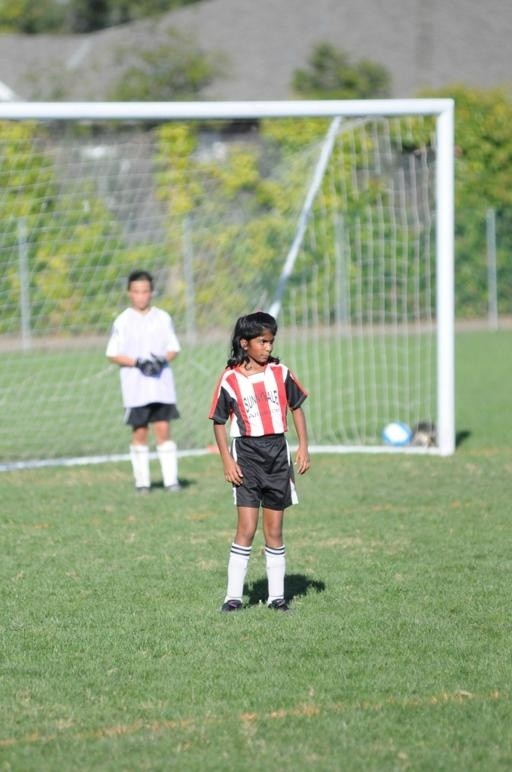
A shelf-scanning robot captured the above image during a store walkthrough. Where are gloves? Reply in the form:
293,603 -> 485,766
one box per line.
136,358 -> 155,377
151,353 -> 168,378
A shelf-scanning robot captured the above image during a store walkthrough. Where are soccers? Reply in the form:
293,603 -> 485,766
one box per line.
383,422 -> 411,446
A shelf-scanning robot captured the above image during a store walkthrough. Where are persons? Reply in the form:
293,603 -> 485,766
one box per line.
210,311 -> 314,612
105,270 -> 185,495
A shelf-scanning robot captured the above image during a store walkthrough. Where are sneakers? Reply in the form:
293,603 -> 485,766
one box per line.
264,599 -> 293,618
136,486 -> 152,496
222,598 -> 245,615
164,483 -> 182,494
